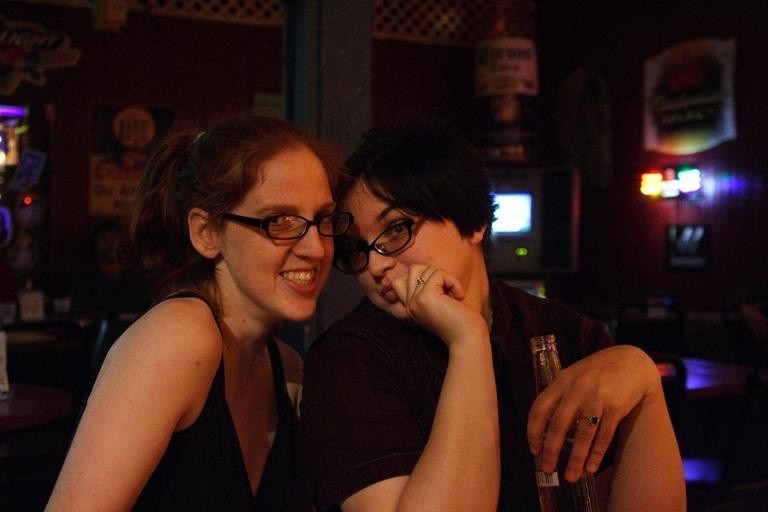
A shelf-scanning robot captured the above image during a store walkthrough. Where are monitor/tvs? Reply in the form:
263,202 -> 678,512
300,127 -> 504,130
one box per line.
487,190 -> 536,240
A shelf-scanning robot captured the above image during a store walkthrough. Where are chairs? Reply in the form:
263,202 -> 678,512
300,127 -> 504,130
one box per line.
0,314 -> 108,437
575,284 -> 766,510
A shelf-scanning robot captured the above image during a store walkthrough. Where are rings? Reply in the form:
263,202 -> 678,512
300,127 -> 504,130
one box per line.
577,413 -> 604,426
416,276 -> 425,287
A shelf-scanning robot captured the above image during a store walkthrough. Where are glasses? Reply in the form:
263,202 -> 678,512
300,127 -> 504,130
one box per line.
332,214 -> 423,275
212,211 -> 353,240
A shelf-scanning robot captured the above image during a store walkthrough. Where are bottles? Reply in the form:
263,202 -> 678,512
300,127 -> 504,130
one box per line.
527,334 -> 609,512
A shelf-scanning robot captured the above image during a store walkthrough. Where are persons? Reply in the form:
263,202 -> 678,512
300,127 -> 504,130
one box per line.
42,115 -> 356,510
287,125 -> 689,510
